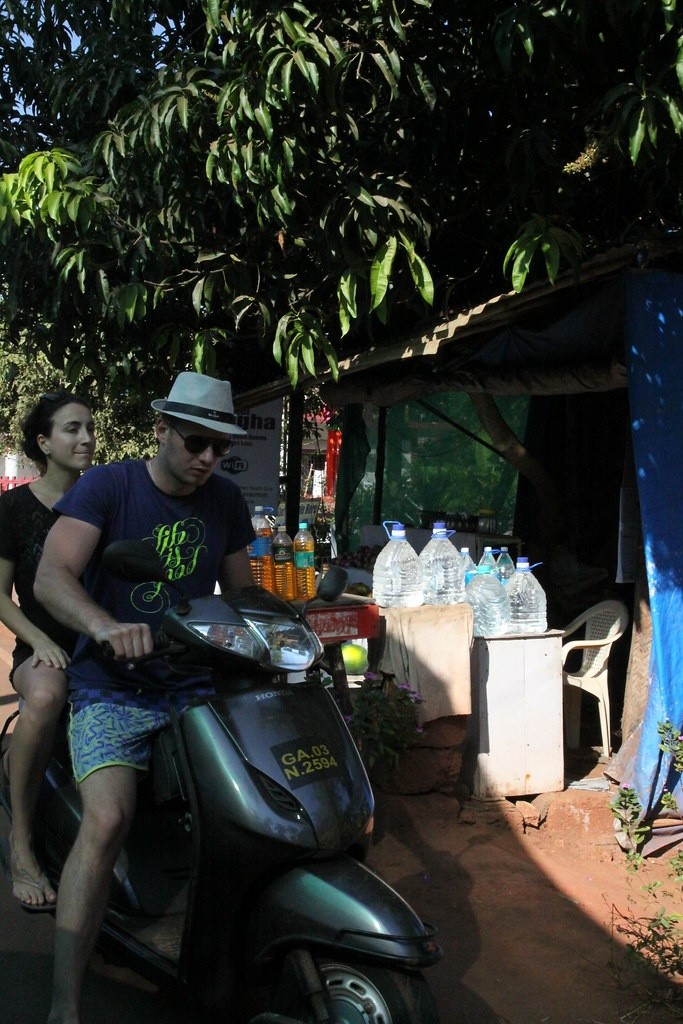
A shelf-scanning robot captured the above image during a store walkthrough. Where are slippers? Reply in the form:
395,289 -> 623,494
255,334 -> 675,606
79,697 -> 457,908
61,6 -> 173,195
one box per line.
7,846 -> 59,912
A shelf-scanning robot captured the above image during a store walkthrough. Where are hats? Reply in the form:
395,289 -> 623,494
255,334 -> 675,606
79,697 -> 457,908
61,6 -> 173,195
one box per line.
151,371 -> 248,435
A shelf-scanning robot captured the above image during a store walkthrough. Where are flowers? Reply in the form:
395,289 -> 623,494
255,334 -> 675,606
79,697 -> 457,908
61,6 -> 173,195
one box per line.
345,668 -> 429,748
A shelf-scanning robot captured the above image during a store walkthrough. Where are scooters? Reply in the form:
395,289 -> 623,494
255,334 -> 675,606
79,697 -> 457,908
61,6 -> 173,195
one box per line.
0,539 -> 445,1023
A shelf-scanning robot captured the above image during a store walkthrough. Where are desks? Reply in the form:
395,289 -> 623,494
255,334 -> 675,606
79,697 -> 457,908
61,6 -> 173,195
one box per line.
475,625 -> 569,800
288,593 -> 379,715
356,524 -> 523,567
353,603 -> 474,725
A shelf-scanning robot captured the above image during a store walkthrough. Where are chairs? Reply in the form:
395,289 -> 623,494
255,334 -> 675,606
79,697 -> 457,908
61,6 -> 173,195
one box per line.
548,600 -> 629,757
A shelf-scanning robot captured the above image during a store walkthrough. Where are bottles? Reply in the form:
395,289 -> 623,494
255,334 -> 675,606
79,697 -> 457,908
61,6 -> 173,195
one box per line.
247,506 -> 274,592
293,523 -> 316,599
272,526 -> 294,601
372,521 -> 548,637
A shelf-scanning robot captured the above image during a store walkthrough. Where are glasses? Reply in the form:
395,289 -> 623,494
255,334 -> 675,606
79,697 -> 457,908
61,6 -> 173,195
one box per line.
164,418 -> 235,457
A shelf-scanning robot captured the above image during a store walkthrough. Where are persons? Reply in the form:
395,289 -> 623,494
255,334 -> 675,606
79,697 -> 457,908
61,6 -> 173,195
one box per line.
33,372 -> 258,1024
0,392 -> 96,911
